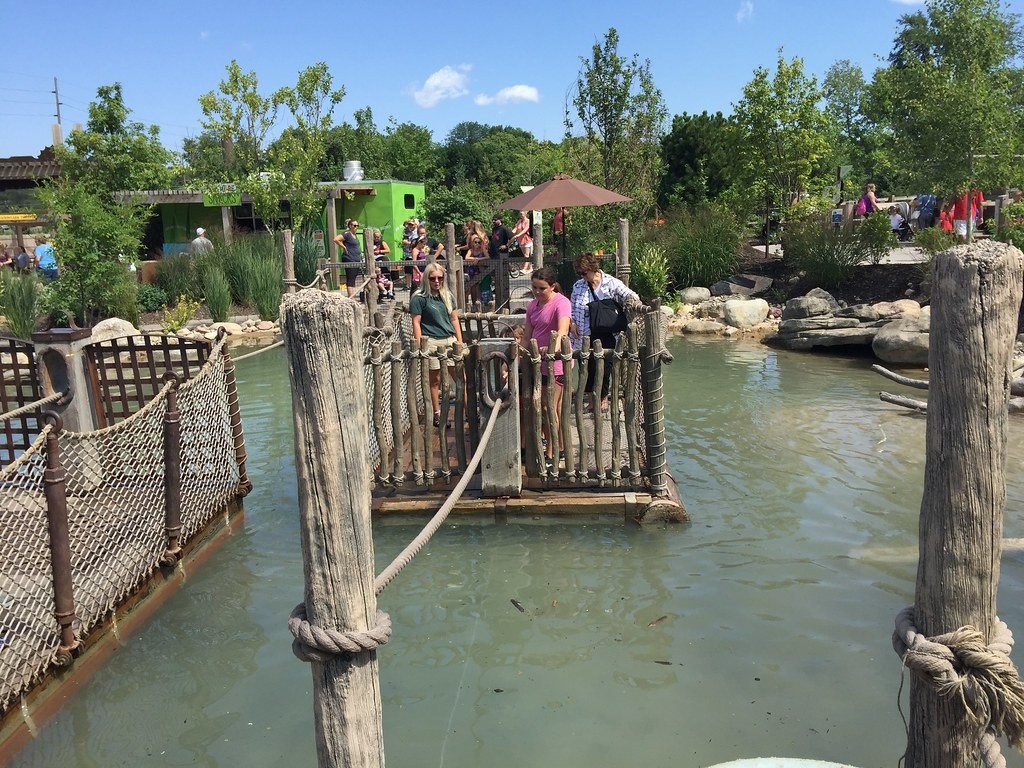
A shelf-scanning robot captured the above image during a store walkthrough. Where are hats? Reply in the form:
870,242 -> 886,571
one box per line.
494,214 -> 503,219
408,219 -> 419,227
196,228 -> 206,236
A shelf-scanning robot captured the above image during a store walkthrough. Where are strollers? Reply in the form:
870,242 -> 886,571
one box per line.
359,254 -> 396,303
894,202 -> 920,241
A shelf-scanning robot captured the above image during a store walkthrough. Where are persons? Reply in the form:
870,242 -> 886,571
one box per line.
190,228 -> 215,254
1007,189 -> 1024,225
401,217 -> 445,301
0,234 -> 59,285
889,206 -> 914,242
334,217 -> 395,300
910,193 -> 937,230
554,207 -> 571,250
456,212 -> 533,305
409,262 -> 481,428
941,188 -> 984,239
500,253 -> 639,464
856,184 -> 880,217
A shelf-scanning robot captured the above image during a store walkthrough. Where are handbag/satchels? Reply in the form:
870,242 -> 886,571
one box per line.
25,269 -> 32,275
586,281 -> 628,333
513,240 -> 519,247
553,234 -> 566,245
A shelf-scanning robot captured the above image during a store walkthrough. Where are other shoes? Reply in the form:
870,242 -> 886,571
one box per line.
465,411 -> 480,422
519,268 -> 533,275
383,289 -> 388,295
521,439 -> 566,467
433,415 -> 451,428
403,286 -> 411,290
388,290 -> 393,296
601,406 -> 609,413
583,405 -> 594,414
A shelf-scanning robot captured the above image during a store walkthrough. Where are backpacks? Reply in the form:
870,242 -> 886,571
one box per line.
856,196 -> 867,216
521,214 -> 533,238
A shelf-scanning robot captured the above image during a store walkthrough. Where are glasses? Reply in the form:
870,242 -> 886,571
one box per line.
475,241 -> 480,244
428,276 -> 444,282
492,219 -> 499,221
577,271 -> 587,277
351,224 -> 359,227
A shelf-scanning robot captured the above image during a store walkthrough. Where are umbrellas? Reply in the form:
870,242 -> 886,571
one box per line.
494,173 -> 633,257
